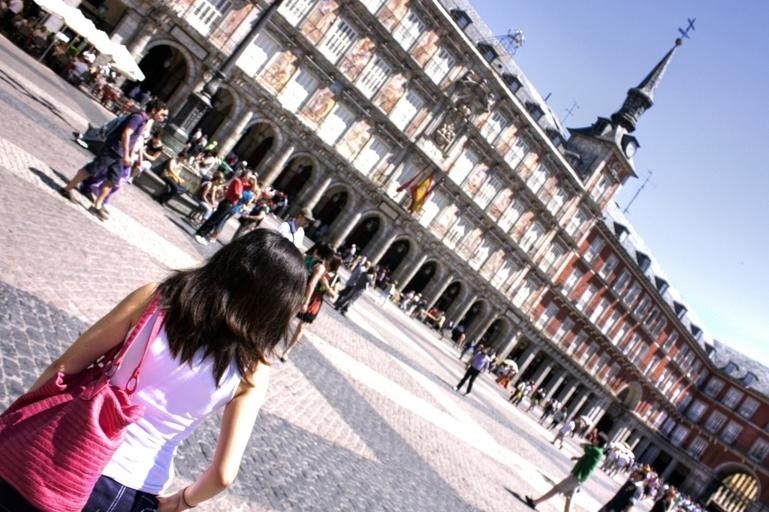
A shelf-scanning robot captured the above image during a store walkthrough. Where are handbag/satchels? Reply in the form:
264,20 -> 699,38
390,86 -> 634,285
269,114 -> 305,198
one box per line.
320,273 -> 341,306
0,345 -> 145,512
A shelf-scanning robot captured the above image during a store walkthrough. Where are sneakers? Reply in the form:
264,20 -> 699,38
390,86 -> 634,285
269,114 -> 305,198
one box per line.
280,357 -> 288,364
525,494 -> 537,510
451,386 -> 472,398
59,181 -> 111,223
194,232 -> 208,247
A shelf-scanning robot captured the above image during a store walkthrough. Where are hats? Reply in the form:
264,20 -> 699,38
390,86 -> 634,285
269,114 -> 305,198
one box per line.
299,208 -> 316,222
